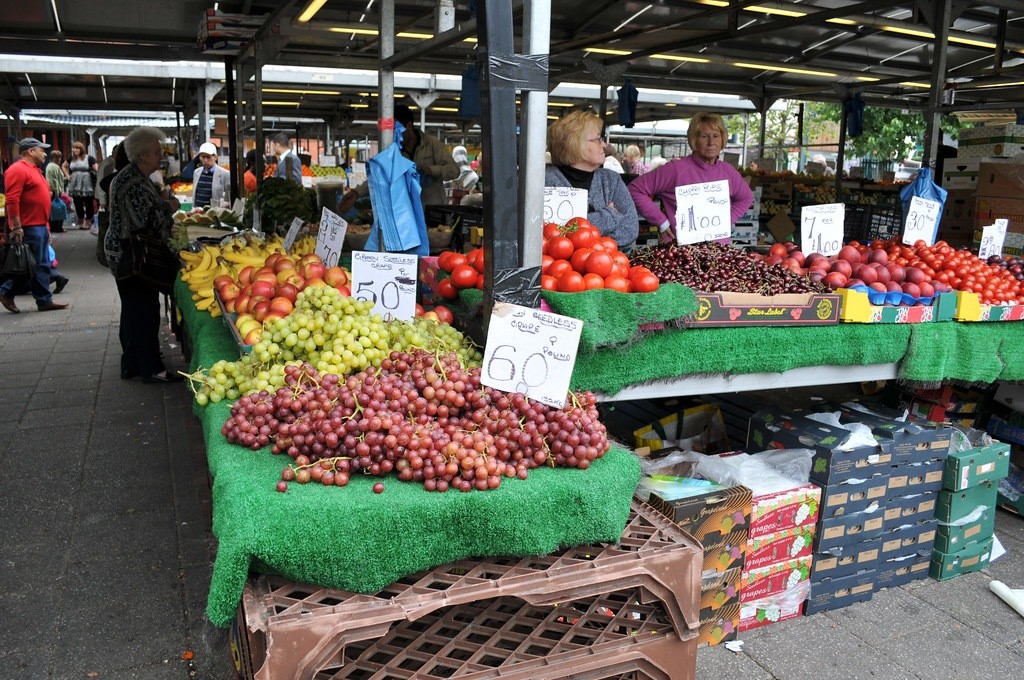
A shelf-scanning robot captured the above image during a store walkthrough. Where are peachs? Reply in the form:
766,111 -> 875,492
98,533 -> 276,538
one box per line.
748,241 -> 946,306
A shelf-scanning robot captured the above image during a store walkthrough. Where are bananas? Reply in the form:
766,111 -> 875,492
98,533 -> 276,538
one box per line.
178,231 -> 316,318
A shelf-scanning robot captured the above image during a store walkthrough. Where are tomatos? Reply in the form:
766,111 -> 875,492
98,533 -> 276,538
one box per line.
991,266 -> 1024,306
849,234 -> 997,307
435,246 -> 485,298
541,217 -> 660,293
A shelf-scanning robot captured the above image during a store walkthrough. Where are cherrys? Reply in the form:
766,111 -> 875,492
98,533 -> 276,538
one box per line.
628,238 -> 833,295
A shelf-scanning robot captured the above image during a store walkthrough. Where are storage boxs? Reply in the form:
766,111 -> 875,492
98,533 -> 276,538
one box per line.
937,124 -> 1024,256
197,8 -> 268,55
731,174 -> 909,242
541,289 -> 1023,649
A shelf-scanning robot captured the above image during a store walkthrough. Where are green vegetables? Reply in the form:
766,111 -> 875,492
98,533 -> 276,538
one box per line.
249,176 -> 318,228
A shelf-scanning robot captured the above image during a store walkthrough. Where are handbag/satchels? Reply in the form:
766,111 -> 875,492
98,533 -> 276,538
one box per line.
50,197 -> 67,221
129,207 -> 176,294
1,243 -> 32,275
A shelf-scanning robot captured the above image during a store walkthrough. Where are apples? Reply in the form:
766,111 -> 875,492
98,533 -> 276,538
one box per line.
309,165 -> 347,180
212,254 -> 454,344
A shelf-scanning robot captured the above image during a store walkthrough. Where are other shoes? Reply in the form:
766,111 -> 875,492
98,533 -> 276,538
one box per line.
78,218 -> 91,229
0,294 -> 20,313
38,302 -> 68,311
53,276 -> 68,293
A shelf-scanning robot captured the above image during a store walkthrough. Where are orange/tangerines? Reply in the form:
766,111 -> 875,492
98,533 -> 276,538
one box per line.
302,165 -> 315,176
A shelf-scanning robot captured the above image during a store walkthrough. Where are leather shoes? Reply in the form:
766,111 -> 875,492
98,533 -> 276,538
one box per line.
121,369 -> 143,379
143,370 -> 184,384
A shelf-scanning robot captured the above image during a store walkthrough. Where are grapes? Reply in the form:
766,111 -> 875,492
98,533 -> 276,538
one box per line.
191,285 -> 611,495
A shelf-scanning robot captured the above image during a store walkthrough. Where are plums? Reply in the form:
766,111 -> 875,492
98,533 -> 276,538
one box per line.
979,255 -> 1024,281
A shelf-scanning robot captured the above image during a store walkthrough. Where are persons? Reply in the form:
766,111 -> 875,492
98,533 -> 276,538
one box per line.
0,138 -> 69,313
337,103 -> 461,214
243,148 -> 267,193
625,111 -> 753,249
601,141 -> 668,174
443,145 -> 474,186
545,110 -> 639,249
94,126 -> 181,384
470,154 -> 482,192
61,142 -> 99,230
272,133 -> 303,186
921,127 -> 958,187
44,149 -> 65,233
804,154 -> 834,179
192,142 -> 230,210
748,159 -> 759,172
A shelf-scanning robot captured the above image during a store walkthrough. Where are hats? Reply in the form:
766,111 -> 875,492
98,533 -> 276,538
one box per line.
19,138 -> 51,155
452,146 -> 468,165
197,142 -> 217,156
